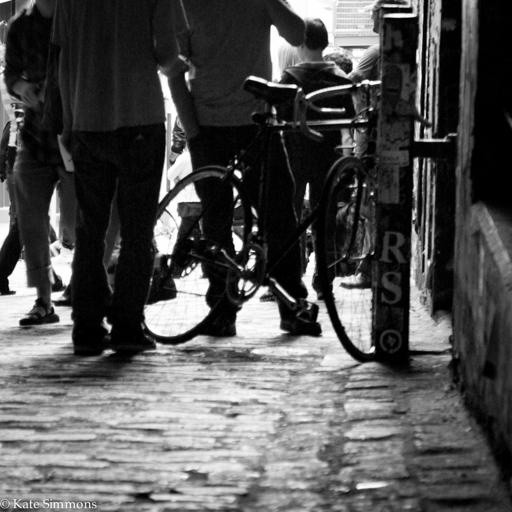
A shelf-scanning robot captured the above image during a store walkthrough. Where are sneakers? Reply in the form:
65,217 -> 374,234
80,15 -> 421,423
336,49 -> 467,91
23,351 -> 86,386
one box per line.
280,317 -> 322,335
201,317 -> 236,337
51,277 -> 71,305
75,332 -> 157,356
145,275 -> 175,305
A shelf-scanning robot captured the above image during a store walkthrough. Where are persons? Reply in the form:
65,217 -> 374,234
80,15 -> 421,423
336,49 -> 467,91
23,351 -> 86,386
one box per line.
271,17 -> 381,299
0,0 -> 179,356
166,0 -> 321,338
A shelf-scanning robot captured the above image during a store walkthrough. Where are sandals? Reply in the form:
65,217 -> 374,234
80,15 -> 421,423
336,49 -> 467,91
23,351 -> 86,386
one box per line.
18,297 -> 59,324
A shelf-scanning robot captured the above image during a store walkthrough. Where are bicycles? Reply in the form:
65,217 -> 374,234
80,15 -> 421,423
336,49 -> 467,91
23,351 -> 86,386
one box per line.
140,71 -> 383,365
